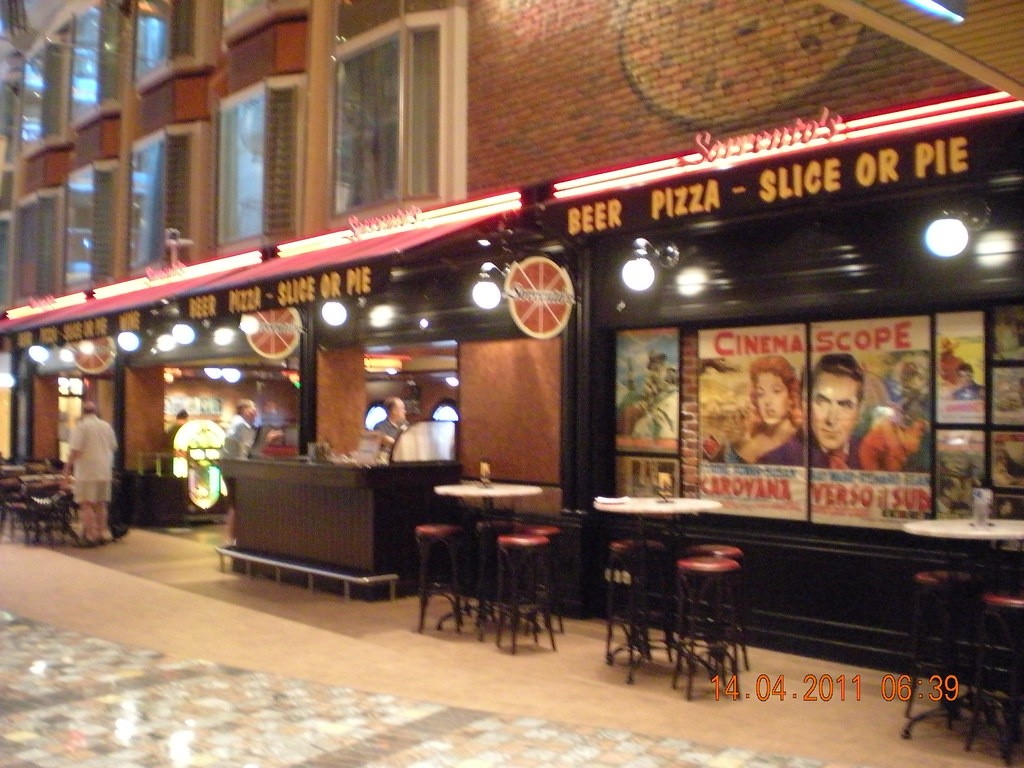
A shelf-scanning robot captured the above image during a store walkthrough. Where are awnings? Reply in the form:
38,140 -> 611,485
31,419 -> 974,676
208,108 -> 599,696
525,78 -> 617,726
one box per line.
0,211 -> 524,354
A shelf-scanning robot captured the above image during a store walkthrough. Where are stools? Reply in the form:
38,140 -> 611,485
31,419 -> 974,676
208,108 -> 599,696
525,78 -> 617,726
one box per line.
899,569 -> 975,715
604,537 -> 751,701
960,591 -> 1024,764
417,517 -> 563,656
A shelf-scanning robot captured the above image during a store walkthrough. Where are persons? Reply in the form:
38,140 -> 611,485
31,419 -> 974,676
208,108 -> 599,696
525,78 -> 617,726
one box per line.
374,397 -> 407,440
167,410 -> 188,448
219,399 -> 256,544
63,402 -> 117,542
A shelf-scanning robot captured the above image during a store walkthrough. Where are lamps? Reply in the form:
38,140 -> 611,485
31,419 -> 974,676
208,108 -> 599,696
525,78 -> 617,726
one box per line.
472,257 -> 510,310
925,197 -> 1019,270
622,235 -> 708,299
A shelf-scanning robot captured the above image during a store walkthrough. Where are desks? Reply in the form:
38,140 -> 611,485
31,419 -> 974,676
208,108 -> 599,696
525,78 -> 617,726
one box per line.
433,482 -> 563,641
900,521 -> 1024,752
592,494 -> 721,681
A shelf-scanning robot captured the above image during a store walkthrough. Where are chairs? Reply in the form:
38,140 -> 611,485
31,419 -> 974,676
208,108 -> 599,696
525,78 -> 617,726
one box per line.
0,474 -> 82,547
